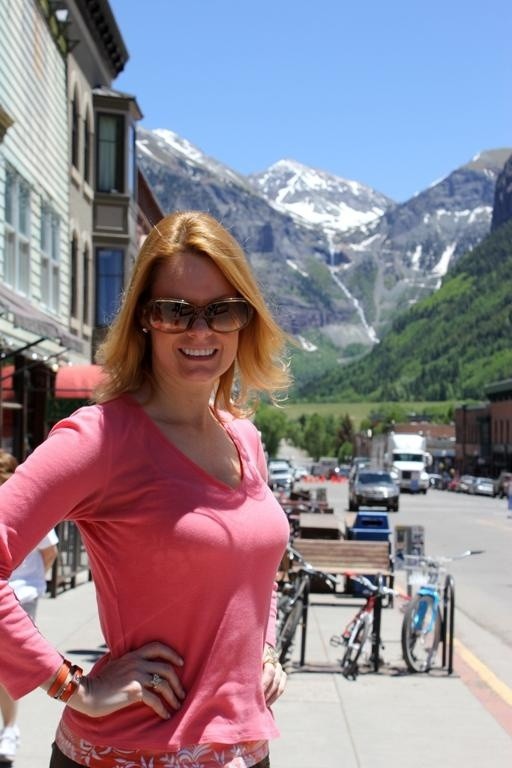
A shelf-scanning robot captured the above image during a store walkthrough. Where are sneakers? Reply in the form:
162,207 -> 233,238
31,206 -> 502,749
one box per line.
0,724 -> 18,763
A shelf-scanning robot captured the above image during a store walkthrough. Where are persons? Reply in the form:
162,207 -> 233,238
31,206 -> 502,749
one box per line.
0,450 -> 60,763
0,211 -> 291,768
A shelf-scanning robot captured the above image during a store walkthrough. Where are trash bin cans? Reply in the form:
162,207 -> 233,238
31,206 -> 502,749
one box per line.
350,512 -> 392,597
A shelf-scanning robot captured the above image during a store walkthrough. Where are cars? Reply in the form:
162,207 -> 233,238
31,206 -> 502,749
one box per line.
267,458 -> 512,499
347,459 -> 400,510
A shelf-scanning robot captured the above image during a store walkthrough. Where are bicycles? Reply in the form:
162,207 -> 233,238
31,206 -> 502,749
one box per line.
393,548 -> 486,674
274,536 -> 339,671
329,570 -> 411,681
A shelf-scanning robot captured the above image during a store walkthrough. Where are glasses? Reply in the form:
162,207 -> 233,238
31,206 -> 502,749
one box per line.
147,297 -> 254,333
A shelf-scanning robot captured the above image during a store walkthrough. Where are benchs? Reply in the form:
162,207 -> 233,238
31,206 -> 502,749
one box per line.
288,538 -> 395,606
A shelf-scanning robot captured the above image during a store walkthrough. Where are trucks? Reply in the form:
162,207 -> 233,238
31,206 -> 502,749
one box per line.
384,431 -> 433,494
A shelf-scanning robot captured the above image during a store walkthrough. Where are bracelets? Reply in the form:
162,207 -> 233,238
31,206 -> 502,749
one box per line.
47,660 -> 83,702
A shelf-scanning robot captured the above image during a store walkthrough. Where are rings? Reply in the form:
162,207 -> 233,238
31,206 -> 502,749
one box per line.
263,647 -> 282,664
150,673 -> 162,688
